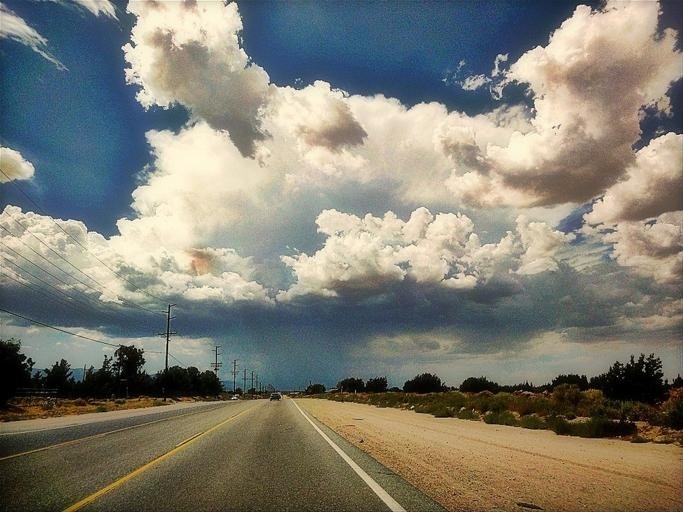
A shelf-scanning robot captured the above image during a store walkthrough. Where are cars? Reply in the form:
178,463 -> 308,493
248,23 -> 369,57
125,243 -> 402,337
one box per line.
269,393 -> 280,401
231,397 -> 237,400
